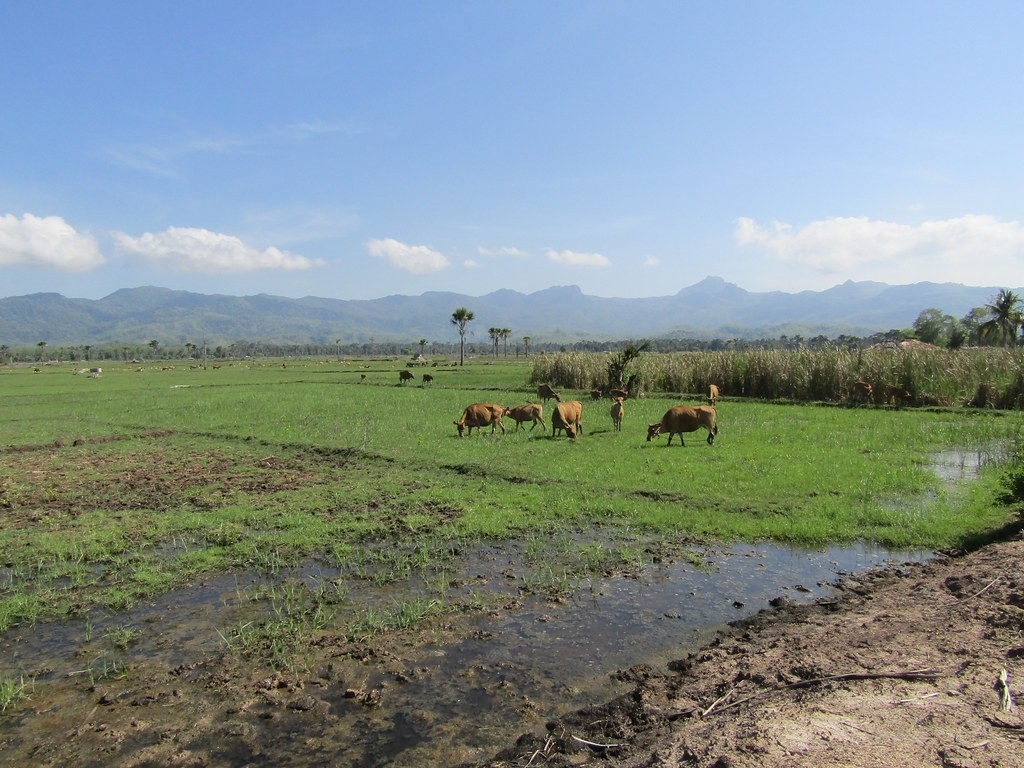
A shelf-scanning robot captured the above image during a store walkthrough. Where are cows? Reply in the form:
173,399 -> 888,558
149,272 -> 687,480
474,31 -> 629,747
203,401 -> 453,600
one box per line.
399,371 -> 415,383
452,381 -> 721,446
422,373 -> 433,386
